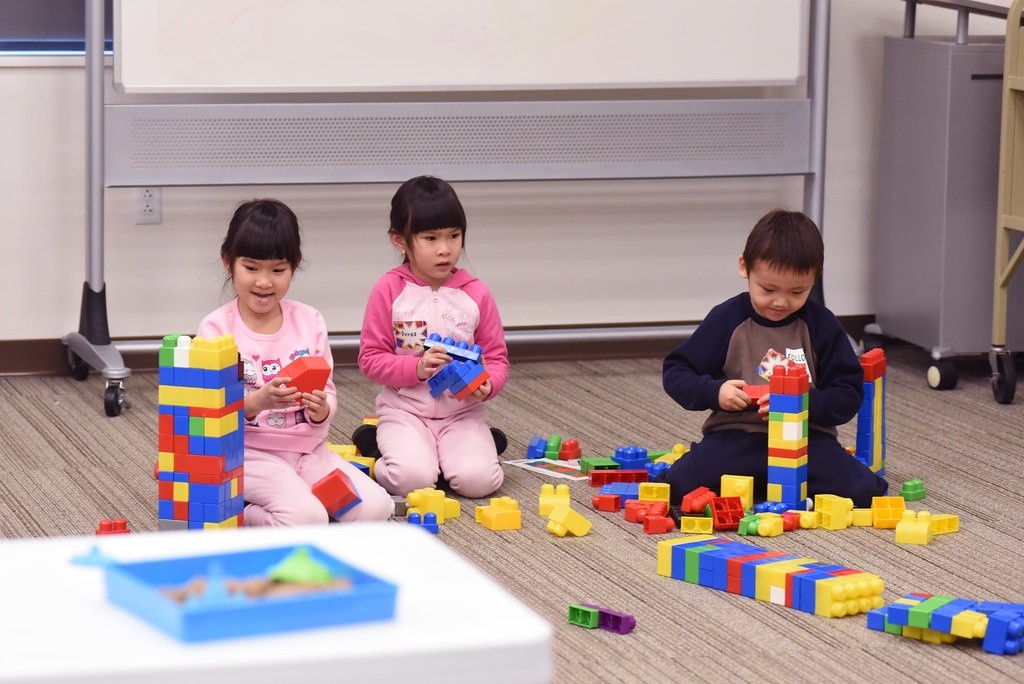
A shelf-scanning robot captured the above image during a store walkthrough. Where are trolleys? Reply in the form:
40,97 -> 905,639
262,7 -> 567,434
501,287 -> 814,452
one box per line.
860,0 -> 1023,389
985,0 -> 1022,404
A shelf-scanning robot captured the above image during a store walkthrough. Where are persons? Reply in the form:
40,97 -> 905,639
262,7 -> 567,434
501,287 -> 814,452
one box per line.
195,199 -> 397,526
662,210 -> 888,508
352,174 -> 510,499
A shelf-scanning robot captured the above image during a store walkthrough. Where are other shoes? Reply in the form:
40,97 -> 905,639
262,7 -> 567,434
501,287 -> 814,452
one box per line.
353,423 -> 382,463
490,427 -> 507,455
